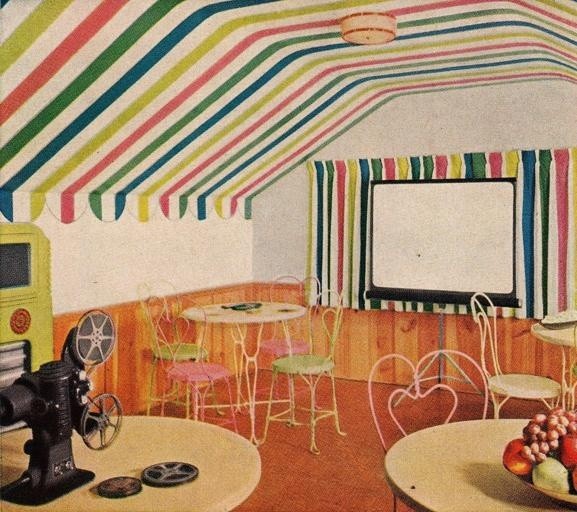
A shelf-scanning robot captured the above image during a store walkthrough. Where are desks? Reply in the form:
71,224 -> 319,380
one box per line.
1,413 -> 264,512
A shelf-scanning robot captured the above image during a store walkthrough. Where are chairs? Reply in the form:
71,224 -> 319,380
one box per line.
134,273 -> 577,512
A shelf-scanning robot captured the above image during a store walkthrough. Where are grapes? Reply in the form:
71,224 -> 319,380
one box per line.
522,407 -> 577,463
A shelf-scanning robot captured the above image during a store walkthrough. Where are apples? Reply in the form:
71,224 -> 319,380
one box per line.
571,466 -> 577,492
561,434 -> 577,467
503,438 -> 532,476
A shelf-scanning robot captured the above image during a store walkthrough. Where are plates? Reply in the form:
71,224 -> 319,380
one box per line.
501,458 -> 576,506
233,303 -> 262,310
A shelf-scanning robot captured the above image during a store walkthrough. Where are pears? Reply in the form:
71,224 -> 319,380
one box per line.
532,458 -> 573,494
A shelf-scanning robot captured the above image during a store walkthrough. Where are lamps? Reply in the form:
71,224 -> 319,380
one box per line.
338,9 -> 400,45
